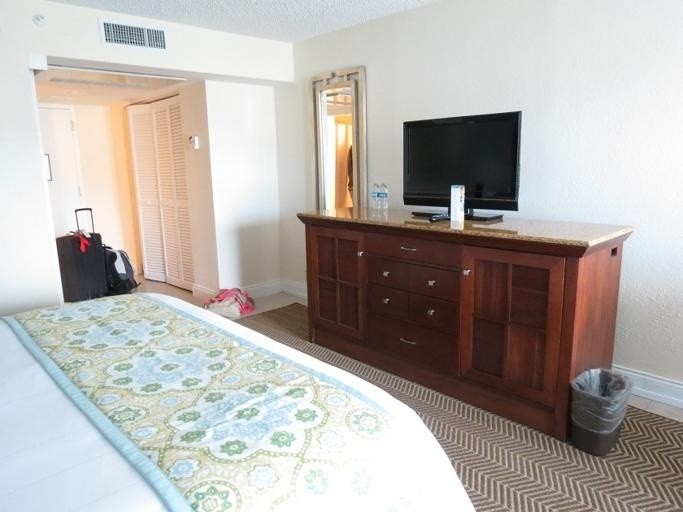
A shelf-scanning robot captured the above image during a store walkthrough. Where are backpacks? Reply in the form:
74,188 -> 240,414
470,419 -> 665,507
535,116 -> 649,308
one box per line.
102,244 -> 139,296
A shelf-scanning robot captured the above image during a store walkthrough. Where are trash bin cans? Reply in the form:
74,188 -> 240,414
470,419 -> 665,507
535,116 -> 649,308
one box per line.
570,367 -> 634,457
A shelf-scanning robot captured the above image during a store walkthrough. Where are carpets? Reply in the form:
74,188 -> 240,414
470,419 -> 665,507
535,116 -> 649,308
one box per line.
232,302 -> 682,512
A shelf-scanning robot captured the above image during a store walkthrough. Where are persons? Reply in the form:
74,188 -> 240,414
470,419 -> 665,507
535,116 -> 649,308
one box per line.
347,146 -> 353,204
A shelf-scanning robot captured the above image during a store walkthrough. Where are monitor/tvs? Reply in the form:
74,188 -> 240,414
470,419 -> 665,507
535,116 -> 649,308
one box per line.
402,111 -> 522,221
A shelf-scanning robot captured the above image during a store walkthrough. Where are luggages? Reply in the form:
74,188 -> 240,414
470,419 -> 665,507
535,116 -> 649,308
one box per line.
56,207 -> 108,303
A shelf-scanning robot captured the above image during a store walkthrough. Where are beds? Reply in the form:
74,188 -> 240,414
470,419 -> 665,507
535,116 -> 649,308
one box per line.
0,292 -> 477,512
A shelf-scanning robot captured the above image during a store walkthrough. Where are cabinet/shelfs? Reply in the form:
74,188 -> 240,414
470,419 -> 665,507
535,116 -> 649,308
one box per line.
297,212 -> 366,363
459,229 -> 635,442
363,223 -> 463,401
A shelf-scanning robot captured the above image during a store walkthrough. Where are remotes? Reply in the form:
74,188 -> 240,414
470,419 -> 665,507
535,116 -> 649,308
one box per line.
428,214 -> 450,222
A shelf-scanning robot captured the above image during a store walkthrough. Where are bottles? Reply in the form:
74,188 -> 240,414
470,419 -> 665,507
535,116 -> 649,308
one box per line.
378,184 -> 388,208
371,184 -> 379,208
